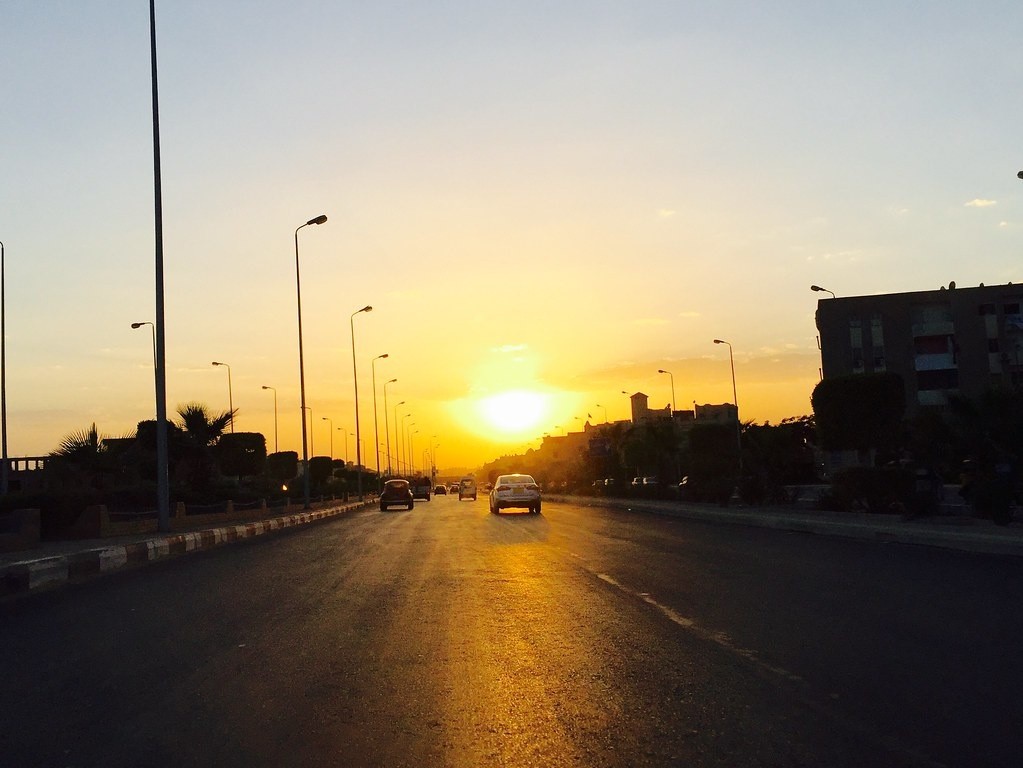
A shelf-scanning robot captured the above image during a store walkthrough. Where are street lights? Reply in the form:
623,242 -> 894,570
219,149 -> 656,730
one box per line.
350,304 -> 373,502
810,285 -> 837,299
263,385 -> 279,453
714,339 -> 746,470
383,378 -> 438,480
322,417 -> 367,469
131,320 -> 157,394
544,403 -> 609,436
301,405 -> 314,457
372,353 -> 390,498
658,369 -> 676,411
212,361 -> 233,434
292,214 -> 329,511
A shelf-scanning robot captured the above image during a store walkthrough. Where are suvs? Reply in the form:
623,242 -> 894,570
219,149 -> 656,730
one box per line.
379,479 -> 414,512
488,474 -> 543,515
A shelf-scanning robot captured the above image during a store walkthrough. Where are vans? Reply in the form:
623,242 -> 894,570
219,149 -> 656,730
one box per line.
456,477 -> 478,501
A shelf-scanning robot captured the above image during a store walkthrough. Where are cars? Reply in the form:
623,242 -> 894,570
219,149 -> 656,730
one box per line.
539,475 -> 700,497
448,481 -> 462,494
434,484 -> 448,496
475,481 -> 495,496
410,485 -> 431,502
